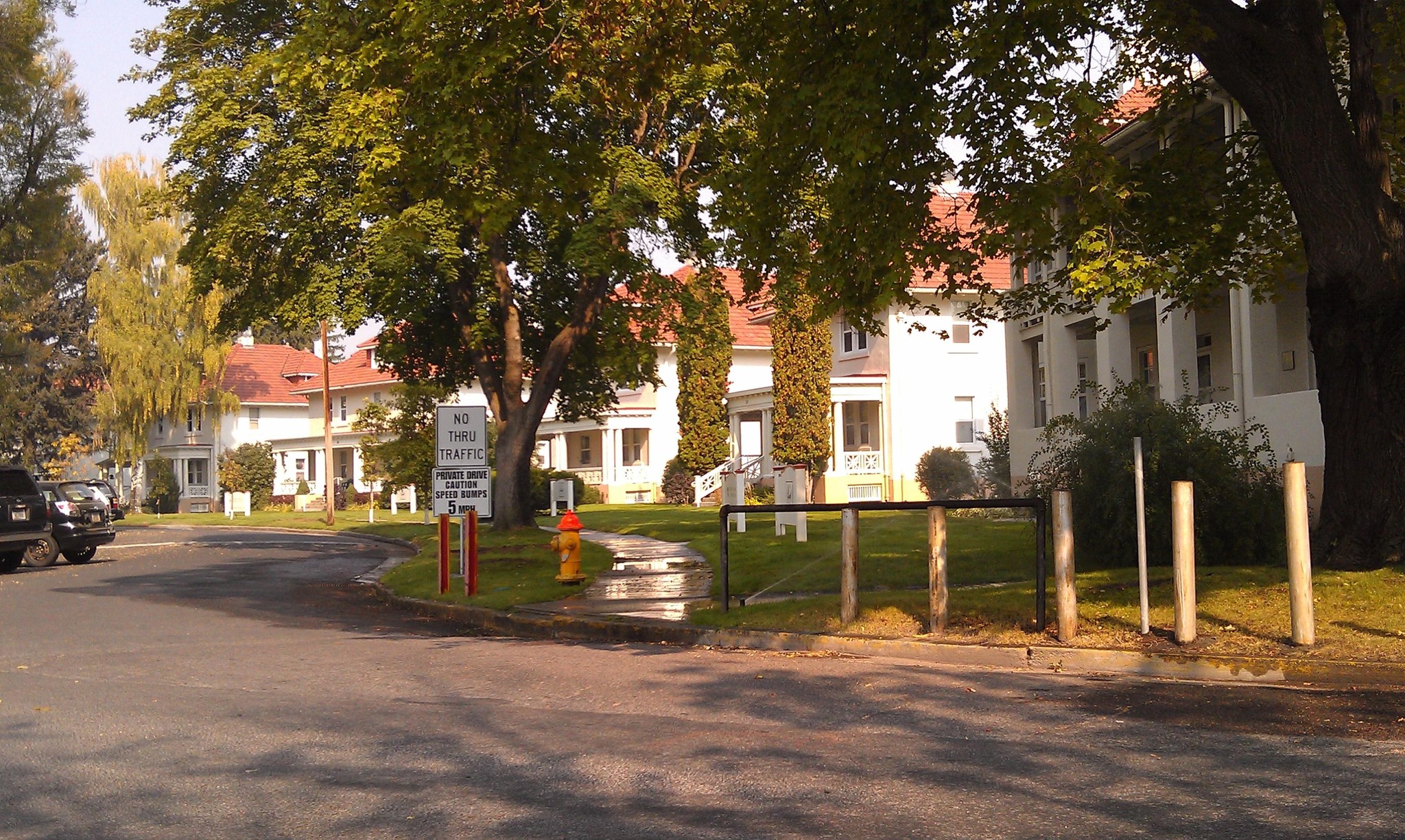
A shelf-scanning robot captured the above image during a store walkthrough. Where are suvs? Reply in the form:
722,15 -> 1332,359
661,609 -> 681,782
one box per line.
0,463 -> 127,573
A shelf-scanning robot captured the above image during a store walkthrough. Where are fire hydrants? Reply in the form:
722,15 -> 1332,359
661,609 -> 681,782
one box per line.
548,509 -> 586,584
